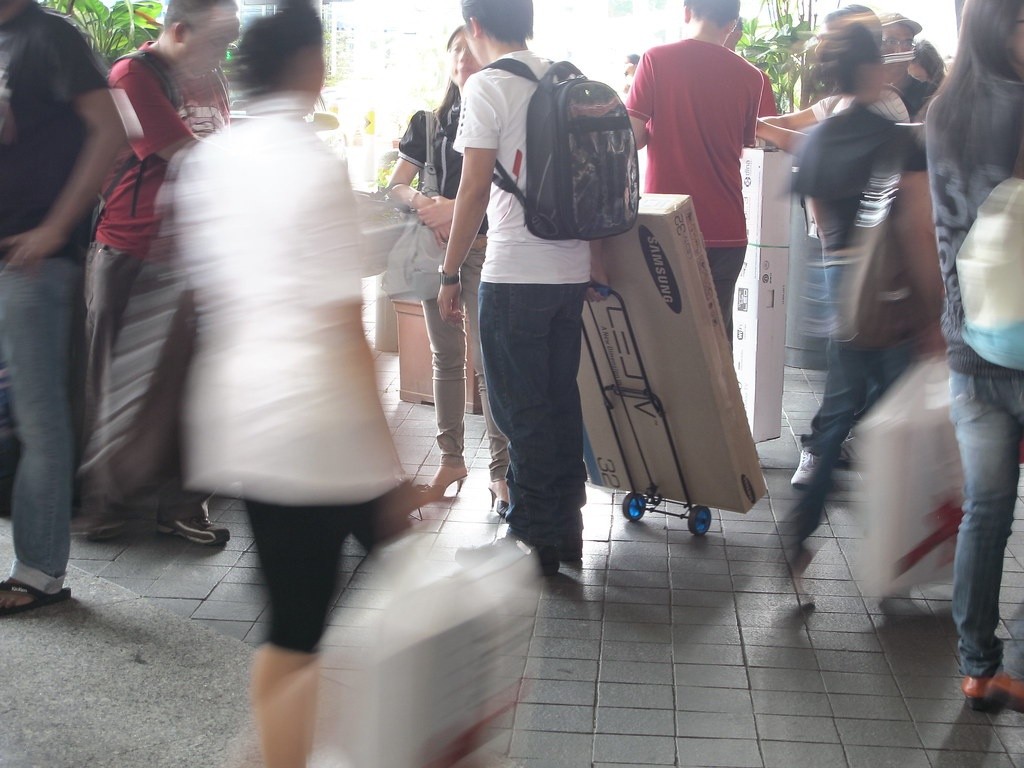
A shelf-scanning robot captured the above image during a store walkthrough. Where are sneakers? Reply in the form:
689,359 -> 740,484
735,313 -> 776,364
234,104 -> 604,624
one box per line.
835,432 -> 854,466
158,512 -> 230,546
87,512 -> 128,542
791,449 -> 824,488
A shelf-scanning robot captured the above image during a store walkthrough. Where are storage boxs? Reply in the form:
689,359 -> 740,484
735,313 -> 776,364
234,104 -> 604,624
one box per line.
727,147 -> 794,444
569,194 -> 769,513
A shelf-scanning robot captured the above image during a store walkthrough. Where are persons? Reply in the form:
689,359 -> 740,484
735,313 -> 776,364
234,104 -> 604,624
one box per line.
387,25 -> 509,520
622,0 -> 777,349
83,0 -> 240,545
436,0 -> 609,577
763,5 -> 944,609
926,0 -> 1024,713
0,0 -> 125,614
170,0 -> 439,768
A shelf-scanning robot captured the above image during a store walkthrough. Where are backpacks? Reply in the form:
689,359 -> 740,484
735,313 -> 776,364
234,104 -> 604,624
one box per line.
473,58 -> 640,240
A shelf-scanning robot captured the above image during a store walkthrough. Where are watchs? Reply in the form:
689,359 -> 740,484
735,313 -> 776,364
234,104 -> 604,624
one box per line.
438,265 -> 460,286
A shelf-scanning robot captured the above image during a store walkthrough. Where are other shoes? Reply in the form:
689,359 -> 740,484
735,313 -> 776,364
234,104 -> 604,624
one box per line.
501,529 -> 585,576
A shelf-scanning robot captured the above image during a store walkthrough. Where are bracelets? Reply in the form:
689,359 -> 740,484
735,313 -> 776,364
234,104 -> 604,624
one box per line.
409,191 -> 421,211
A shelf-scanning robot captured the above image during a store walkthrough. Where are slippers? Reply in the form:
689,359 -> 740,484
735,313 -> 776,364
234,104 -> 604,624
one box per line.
0,581 -> 71,615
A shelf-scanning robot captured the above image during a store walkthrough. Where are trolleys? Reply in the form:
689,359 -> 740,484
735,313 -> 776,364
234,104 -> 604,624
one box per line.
577,283 -> 713,537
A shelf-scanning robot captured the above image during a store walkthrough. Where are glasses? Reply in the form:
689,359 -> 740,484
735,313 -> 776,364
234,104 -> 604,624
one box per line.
881,37 -> 915,51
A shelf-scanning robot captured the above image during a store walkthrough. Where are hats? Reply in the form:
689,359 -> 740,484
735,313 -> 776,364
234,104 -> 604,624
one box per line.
880,13 -> 923,36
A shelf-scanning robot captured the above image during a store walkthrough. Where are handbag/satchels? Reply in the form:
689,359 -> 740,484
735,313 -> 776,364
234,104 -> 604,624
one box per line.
387,111 -> 446,300
854,354 -> 965,593
307,523 -> 538,768
956,169 -> 1024,368
845,147 -> 936,352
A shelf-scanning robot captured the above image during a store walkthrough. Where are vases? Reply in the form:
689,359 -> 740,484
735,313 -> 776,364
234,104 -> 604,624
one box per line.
392,297 -> 485,415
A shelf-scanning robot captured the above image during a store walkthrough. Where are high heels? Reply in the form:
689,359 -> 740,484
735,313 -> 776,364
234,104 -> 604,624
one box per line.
413,471 -> 468,503
488,487 -> 509,518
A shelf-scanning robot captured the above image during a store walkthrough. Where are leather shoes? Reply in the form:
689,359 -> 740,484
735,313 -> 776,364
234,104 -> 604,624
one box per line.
963,671 -> 996,709
989,673 -> 1024,714
